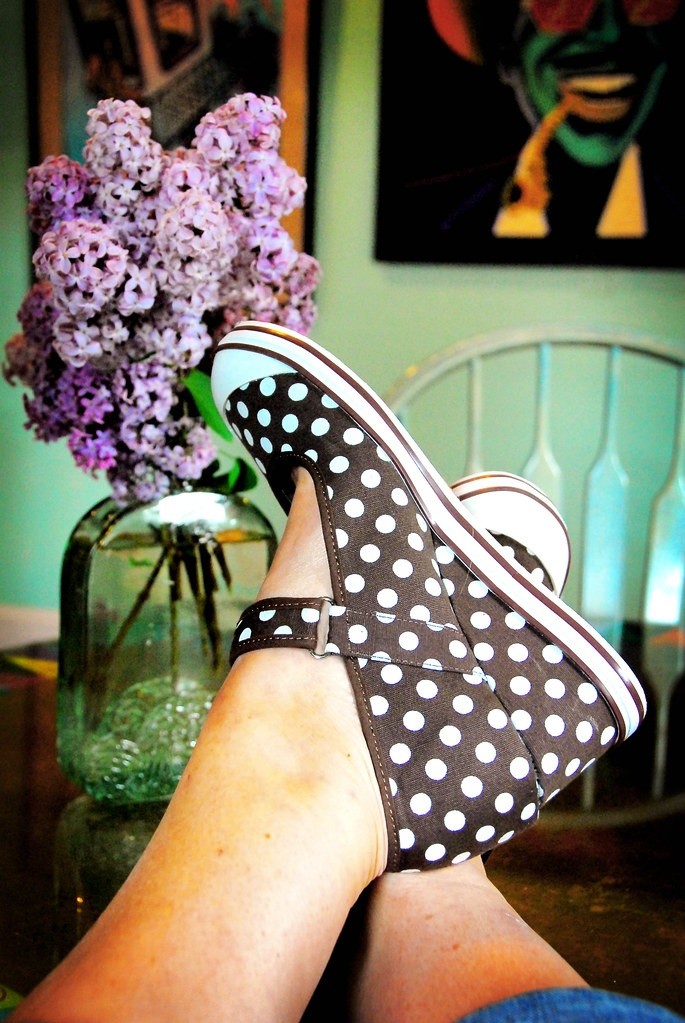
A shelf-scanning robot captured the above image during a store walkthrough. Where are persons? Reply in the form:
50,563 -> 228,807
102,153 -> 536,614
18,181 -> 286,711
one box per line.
5,321 -> 685,1023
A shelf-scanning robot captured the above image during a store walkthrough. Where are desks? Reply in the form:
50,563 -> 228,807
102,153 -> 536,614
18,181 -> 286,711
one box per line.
0,607 -> 685,1022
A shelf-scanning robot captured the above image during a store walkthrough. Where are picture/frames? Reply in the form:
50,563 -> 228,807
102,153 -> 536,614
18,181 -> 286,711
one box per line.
23,1 -> 323,289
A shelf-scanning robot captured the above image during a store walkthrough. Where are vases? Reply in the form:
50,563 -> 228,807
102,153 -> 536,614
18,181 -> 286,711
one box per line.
54,488 -> 281,807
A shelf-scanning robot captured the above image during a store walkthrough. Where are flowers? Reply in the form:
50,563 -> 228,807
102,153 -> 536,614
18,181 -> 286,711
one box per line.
0,92 -> 322,507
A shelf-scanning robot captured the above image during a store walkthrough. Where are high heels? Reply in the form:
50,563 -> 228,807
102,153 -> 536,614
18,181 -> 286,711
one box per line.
210,320 -> 647,872
